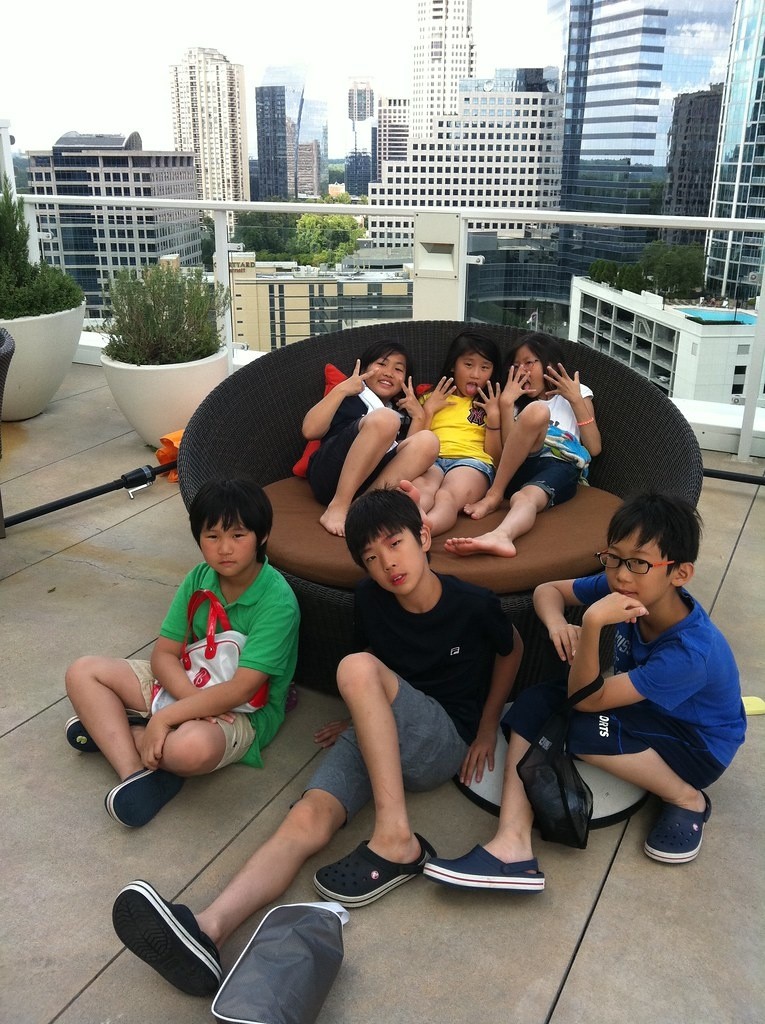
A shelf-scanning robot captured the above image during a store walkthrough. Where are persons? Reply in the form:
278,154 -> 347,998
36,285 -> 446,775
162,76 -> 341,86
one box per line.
63,476 -> 302,831
110,485 -> 525,1000
301,339 -> 439,538
394,331 -> 504,538
445,333 -> 603,559
423,493 -> 749,891
700,295 -> 724,308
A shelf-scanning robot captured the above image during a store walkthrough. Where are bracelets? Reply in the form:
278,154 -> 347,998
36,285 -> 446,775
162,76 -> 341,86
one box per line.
486,425 -> 501,430
577,417 -> 594,426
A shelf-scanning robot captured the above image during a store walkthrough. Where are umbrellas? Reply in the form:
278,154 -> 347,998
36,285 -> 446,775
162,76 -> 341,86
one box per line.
4,427 -> 185,527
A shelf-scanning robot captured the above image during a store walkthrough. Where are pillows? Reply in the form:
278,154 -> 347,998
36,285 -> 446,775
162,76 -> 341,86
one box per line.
294,362 -> 435,480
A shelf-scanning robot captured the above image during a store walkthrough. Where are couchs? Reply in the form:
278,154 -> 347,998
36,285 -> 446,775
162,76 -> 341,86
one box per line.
178,321 -> 705,700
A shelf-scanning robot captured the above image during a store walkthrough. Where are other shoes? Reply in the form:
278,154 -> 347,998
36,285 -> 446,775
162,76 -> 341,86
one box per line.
286,682 -> 298,712
111,879 -> 222,997
65,714 -> 150,752
644,790 -> 712,863
314,830 -> 438,908
104,766 -> 187,828
423,844 -> 545,894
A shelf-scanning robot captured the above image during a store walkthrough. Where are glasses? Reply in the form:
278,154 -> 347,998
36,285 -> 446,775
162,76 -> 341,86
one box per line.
595,549 -> 674,574
514,359 -> 539,371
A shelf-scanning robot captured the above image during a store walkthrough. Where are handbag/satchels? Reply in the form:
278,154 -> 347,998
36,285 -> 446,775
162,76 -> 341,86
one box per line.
516,675 -> 604,849
150,589 -> 269,716
210,902 -> 350,1024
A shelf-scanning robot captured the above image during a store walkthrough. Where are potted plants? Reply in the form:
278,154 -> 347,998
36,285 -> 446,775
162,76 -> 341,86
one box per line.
100,261 -> 232,451
0,171 -> 86,422
